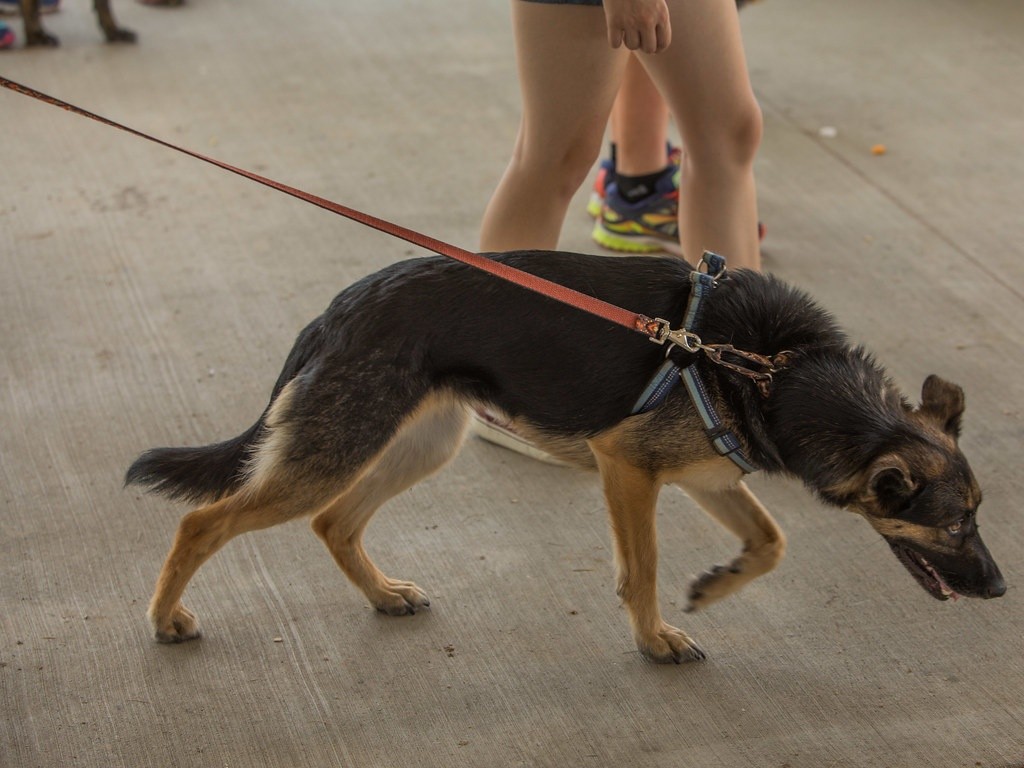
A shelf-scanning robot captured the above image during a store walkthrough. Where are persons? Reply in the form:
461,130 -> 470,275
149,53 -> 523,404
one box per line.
17,0 -> 136,47
474,0 -> 763,469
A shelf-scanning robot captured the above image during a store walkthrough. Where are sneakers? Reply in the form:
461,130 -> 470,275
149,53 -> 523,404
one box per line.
592,166 -> 767,256
587,148 -> 682,216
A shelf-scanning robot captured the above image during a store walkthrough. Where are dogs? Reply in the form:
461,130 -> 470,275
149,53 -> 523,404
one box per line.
15,0 -> 186,49
123,249 -> 1008,664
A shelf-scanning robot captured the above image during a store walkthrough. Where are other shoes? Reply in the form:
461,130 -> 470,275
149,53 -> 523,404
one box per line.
0,23 -> 14,48
25,30 -> 57,46
473,408 -> 568,466
108,30 -> 135,43
